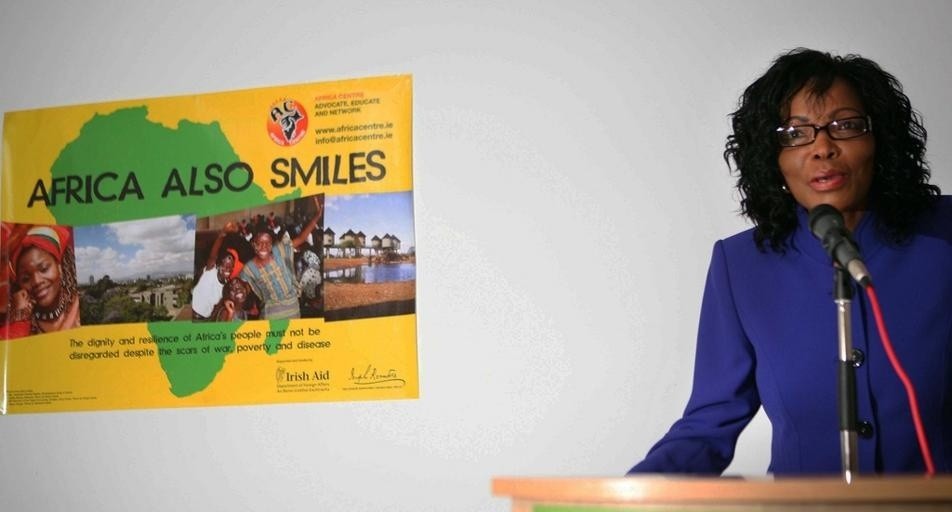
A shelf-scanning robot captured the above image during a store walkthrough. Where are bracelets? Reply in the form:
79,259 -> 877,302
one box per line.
10,304 -> 32,324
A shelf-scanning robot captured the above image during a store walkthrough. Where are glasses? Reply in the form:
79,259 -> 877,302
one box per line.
773,115 -> 873,147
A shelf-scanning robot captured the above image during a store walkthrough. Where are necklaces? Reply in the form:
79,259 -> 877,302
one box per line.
33,293 -> 66,321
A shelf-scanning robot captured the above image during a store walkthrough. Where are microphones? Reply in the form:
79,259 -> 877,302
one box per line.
805,205 -> 871,292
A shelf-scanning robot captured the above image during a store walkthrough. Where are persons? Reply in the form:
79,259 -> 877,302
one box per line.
625,45 -> 952,478
191,195 -> 324,318
0,226 -> 81,342
